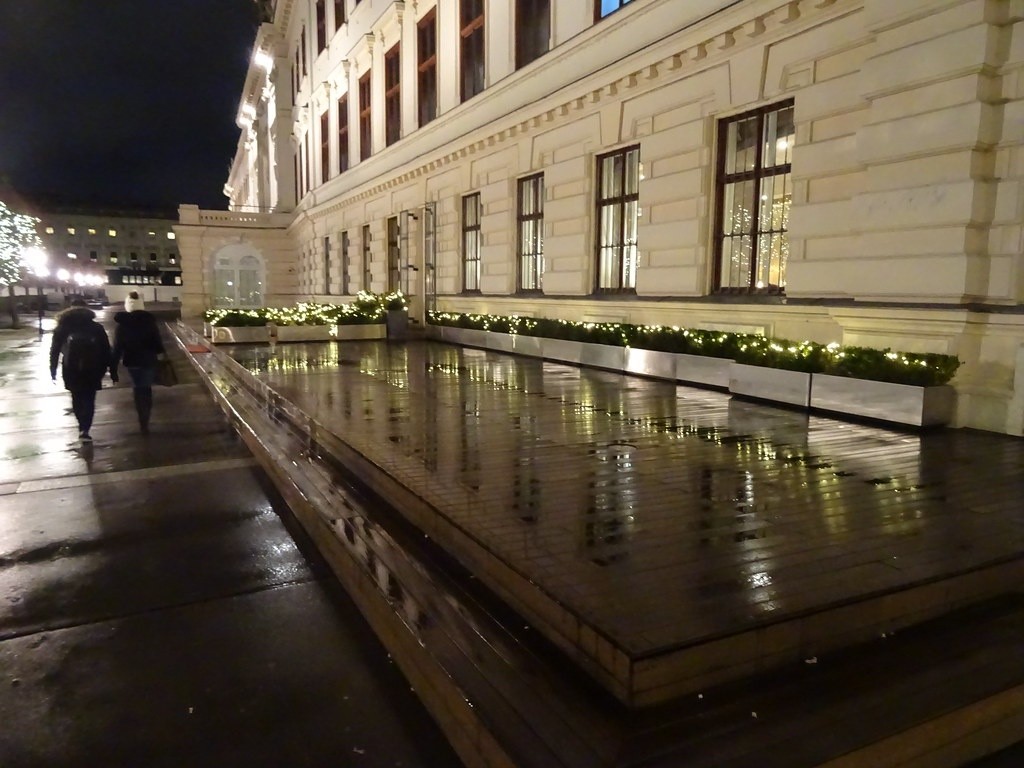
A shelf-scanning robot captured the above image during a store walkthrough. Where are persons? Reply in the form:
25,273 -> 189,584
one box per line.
49,299 -> 111,442
113,290 -> 166,433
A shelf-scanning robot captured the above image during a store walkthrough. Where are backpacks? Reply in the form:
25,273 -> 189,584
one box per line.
59,320 -> 103,375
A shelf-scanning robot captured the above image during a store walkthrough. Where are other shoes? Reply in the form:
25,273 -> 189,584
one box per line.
78,430 -> 92,442
140,424 -> 149,433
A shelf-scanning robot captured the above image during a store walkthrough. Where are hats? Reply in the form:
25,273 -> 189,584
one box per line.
124,291 -> 145,313
72,299 -> 88,308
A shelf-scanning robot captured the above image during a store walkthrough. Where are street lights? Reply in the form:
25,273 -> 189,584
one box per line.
57,269 -> 84,294
27,247 -> 51,339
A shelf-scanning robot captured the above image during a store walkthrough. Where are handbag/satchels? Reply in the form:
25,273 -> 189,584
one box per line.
152,348 -> 178,387
